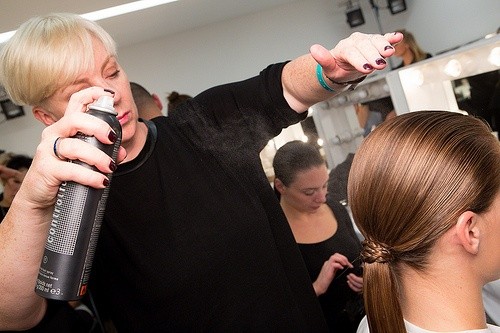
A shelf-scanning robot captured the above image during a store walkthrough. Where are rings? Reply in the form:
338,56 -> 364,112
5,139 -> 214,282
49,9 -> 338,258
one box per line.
54,137 -> 65,160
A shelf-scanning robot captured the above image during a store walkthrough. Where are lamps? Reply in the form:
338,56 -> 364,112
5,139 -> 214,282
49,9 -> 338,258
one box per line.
386,0 -> 407,15
335,0 -> 366,29
0,98 -> 25,120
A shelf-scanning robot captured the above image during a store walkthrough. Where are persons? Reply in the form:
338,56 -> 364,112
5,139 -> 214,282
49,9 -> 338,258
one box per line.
348,110 -> 500,333
0,152 -> 37,225
387,29 -> 434,70
0,13 -> 403,333
270,141 -> 366,333
129,82 -> 169,126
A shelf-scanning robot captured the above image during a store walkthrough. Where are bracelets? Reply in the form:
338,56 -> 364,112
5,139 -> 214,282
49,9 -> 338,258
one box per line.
316,62 -> 371,98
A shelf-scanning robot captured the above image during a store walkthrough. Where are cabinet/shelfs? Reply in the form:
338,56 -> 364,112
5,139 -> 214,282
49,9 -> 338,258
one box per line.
312,34 -> 500,241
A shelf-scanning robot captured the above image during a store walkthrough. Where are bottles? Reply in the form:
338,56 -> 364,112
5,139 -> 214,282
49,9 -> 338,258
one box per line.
34,97 -> 122,300
